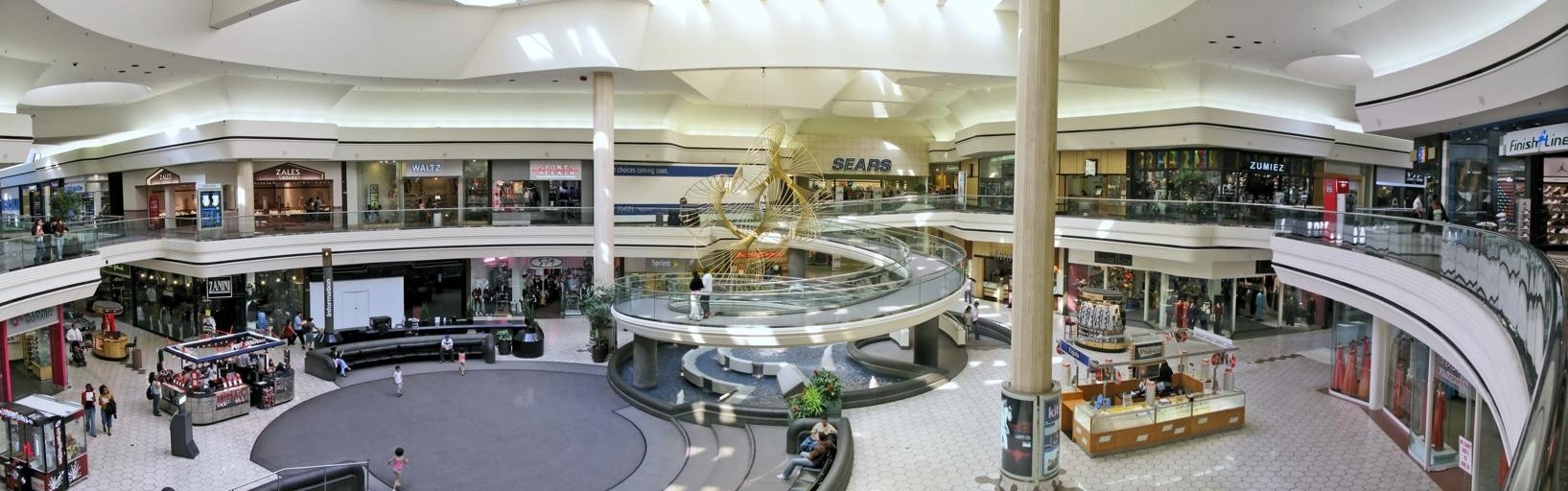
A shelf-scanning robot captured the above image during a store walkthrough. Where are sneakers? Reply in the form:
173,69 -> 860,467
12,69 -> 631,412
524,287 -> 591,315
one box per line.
777,474 -> 788,479
290,342 -> 314,351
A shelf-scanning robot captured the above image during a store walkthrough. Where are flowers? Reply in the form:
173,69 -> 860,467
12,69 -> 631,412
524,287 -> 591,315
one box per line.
788,366 -> 843,419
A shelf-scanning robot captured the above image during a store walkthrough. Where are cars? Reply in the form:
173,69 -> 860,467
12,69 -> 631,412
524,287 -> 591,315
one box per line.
62,309 -> 96,341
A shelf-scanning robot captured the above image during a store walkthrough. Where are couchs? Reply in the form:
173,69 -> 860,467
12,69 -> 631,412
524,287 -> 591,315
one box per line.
785,415 -> 856,491
304,334 -> 494,379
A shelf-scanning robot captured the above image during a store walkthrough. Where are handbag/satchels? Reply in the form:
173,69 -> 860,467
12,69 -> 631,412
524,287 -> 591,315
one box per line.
280,331 -> 287,339
106,400 -> 117,415
146,386 -> 153,400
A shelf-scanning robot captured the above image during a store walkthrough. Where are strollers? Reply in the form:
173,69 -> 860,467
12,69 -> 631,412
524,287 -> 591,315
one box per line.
68,339 -> 87,367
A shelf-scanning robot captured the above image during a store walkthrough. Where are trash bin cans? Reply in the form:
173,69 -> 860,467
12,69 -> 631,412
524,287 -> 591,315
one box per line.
369,316 -> 391,329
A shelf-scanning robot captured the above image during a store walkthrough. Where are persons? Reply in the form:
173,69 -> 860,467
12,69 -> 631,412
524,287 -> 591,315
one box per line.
302,317 -> 320,350
699,268 -> 714,319
237,352 -> 252,384
1426,194 -> 1449,236
33,218 -> 47,266
97,384 -> 114,435
438,334 -> 457,364
1411,193 -> 1424,233
73,345 -> 82,359
1390,189 -> 1418,209
964,274 -> 973,304
303,195 -> 323,222
471,284 -> 483,316
673,197 -> 694,227
1155,292 -> 1223,336
53,218 -> 69,262
391,365 -> 405,396
182,366 -> 192,383
148,372 -> 163,416
1456,160 -> 1482,203
386,446 -> 409,491
970,301 -> 981,340
1285,295 -> 1303,325
1156,360 -> 1174,398
492,180 -> 541,210
329,345 -> 351,378
579,284 -> 588,300
1306,297 -> 1316,331
256,307 -> 281,342
763,265 -> 784,288
1082,190 -> 1087,196
799,415 -> 838,452
1243,285 -> 1253,318
290,310 -> 306,349
418,199 -> 427,223
426,198 -> 433,209
776,431 -> 838,480
280,318 -> 297,346
1101,366 -> 1112,379
256,353 -> 291,382
1119,305 -> 1127,326
156,348 -> 173,383
204,360 -> 221,383
66,322 -> 83,357
80,383 -> 98,438
1254,287 -> 1268,322
42,218 -> 58,262
1333,336 -> 1448,452
457,347 -> 467,375
687,269 -> 705,322
483,286 -> 494,317
961,306 -> 973,340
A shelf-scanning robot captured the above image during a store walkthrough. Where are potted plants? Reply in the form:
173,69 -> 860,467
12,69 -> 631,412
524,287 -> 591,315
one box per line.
465,304 -> 474,324
421,300 -> 431,326
496,330 -> 511,354
579,285 -> 612,363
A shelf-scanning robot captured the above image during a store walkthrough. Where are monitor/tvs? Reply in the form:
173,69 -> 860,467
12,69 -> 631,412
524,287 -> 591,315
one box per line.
1138,364 -> 1160,379
250,355 -> 258,365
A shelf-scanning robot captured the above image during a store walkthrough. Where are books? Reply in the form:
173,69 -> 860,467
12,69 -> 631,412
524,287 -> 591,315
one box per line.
85,391 -> 93,402
